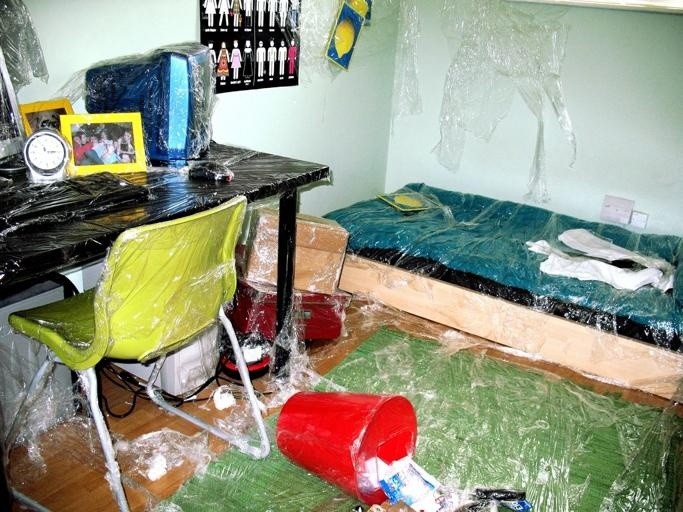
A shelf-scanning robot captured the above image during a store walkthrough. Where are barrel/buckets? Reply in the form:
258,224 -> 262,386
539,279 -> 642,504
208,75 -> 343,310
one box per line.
277,390 -> 417,506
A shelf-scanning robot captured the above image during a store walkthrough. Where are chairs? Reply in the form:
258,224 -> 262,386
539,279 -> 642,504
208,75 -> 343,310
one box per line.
3,195 -> 270,510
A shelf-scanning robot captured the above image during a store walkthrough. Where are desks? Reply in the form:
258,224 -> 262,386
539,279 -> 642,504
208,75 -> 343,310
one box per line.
0,142 -> 330,511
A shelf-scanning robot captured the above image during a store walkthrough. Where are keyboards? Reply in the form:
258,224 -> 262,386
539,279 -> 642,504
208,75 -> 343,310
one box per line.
0,171 -> 149,234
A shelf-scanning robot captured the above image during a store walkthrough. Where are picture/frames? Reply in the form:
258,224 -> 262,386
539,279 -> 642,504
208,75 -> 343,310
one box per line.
18,97 -> 146,179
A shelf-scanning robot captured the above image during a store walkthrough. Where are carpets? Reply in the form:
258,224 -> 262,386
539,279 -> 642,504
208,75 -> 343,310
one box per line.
151,325 -> 683,511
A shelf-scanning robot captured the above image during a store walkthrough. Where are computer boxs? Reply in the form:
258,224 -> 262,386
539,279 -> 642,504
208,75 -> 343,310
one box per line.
110,323 -> 222,396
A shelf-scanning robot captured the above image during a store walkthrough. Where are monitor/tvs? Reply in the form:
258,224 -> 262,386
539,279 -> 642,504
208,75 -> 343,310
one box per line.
0,42 -> 27,160
85,45 -> 214,165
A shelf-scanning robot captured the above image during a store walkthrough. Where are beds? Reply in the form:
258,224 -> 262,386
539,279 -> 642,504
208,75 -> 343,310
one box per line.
320,181 -> 683,403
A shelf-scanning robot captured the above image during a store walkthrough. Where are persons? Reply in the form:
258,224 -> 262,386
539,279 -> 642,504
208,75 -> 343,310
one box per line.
69,125 -> 138,170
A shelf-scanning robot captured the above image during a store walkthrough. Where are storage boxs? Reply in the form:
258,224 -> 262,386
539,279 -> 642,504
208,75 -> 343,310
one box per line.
241,206 -> 350,296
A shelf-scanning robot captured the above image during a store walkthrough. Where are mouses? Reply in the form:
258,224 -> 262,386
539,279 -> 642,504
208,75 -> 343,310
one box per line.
189,162 -> 234,182
